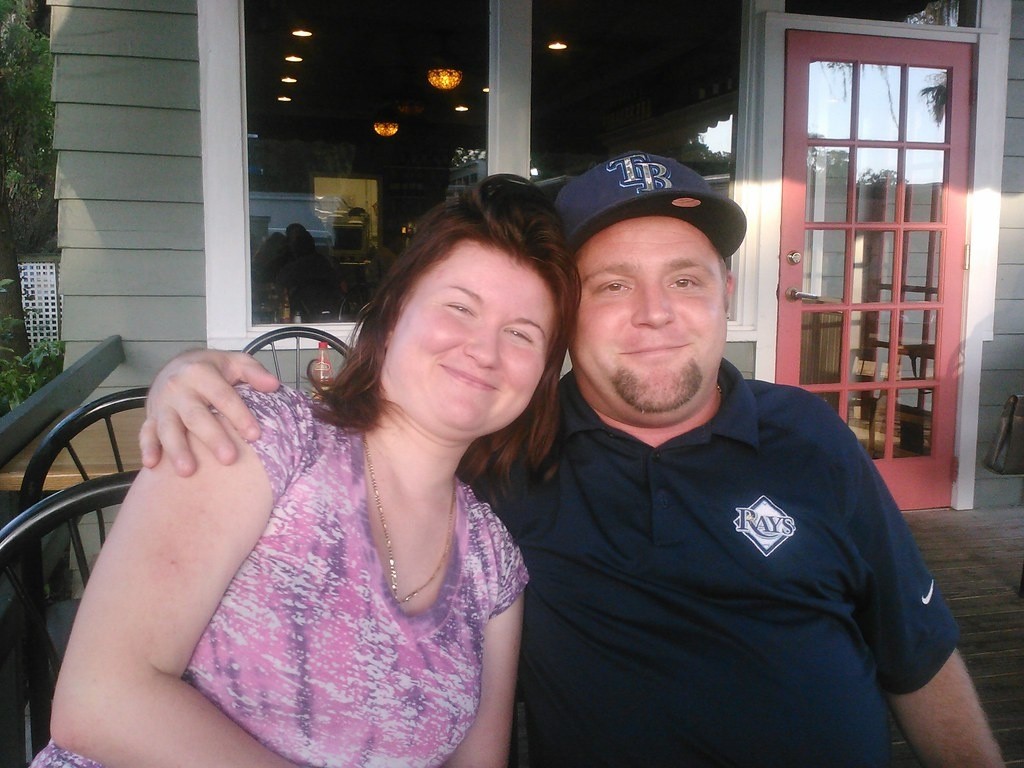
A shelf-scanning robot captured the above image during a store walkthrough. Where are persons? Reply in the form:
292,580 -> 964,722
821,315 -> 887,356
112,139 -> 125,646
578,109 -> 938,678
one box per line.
28,173 -> 582,768
139,152 -> 1007,768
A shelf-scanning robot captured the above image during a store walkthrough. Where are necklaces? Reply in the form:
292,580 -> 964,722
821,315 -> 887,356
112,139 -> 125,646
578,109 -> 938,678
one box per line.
363,430 -> 456,604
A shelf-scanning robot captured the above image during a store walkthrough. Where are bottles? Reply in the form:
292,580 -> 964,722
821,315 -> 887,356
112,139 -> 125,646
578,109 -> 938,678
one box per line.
280,296 -> 291,323
315,342 -> 333,402
293,312 -> 303,323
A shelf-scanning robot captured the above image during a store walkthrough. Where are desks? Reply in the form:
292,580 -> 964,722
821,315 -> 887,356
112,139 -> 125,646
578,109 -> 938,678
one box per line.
1,406 -> 150,492
801,296 -> 846,418
867,334 -> 934,458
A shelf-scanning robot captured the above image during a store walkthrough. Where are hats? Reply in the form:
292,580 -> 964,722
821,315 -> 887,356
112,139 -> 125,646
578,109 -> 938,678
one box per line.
552,150 -> 748,260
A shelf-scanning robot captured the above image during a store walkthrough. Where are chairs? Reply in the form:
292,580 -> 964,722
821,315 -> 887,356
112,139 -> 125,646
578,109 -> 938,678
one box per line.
240,327 -> 355,399
1,469 -> 142,768
19,387 -> 153,768
